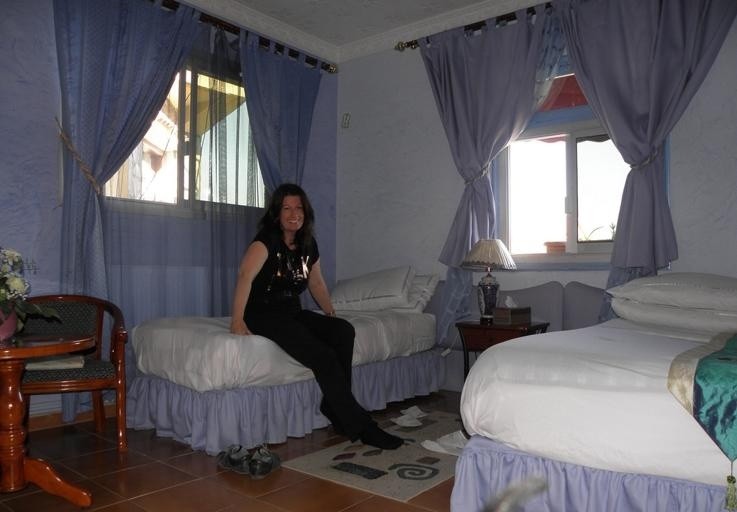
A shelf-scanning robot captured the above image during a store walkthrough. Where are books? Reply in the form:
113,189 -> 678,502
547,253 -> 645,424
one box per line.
24,355 -> 85,371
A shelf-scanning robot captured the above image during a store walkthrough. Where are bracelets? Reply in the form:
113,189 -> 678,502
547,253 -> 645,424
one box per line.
327,310 -> 335,316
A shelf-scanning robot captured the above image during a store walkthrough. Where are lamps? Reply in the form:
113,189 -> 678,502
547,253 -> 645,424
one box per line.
453,236 -> 520,325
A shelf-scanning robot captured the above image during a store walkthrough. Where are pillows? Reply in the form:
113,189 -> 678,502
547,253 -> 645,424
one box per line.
600,271 -> 736,315
608,287 -> 735,338
325,262 -> 417,316
381,270 -> 448,316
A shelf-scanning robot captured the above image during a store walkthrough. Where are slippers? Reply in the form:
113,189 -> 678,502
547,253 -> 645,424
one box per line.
400,406 -> 426,418
390,414 -> 423,427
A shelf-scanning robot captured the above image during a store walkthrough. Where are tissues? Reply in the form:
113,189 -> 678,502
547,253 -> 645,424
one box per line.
492,296 -> 532,325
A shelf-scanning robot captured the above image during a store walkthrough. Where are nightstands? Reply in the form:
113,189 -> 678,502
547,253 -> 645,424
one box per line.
450,311 -> 552,424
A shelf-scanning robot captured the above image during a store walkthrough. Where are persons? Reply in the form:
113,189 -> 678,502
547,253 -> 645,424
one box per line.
230,183 -> 404,450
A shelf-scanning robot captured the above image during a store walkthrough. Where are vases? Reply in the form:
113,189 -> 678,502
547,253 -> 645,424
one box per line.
0,295 -> 20,344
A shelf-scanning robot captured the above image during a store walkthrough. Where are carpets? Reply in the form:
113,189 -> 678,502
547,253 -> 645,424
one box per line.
265,402 -> 472,505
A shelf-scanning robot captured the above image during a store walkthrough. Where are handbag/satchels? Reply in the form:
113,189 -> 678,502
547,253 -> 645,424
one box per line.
25,342 -> 85,370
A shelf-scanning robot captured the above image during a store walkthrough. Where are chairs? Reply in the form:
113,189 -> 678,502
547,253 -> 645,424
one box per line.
8,287 -> 134,464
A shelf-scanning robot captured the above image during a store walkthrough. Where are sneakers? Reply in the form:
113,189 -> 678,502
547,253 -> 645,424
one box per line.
217,445 -> 249,474
249,447 -> 280,478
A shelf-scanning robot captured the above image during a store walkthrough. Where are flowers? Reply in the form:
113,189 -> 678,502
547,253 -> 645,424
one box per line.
0,242 -> 65,335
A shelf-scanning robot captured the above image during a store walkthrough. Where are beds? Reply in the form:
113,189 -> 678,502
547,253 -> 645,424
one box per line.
114,277 -> 457,481
436,306 -> 735,511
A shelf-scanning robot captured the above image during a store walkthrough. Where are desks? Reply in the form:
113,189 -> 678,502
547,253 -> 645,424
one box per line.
1,312 -> 106,512
